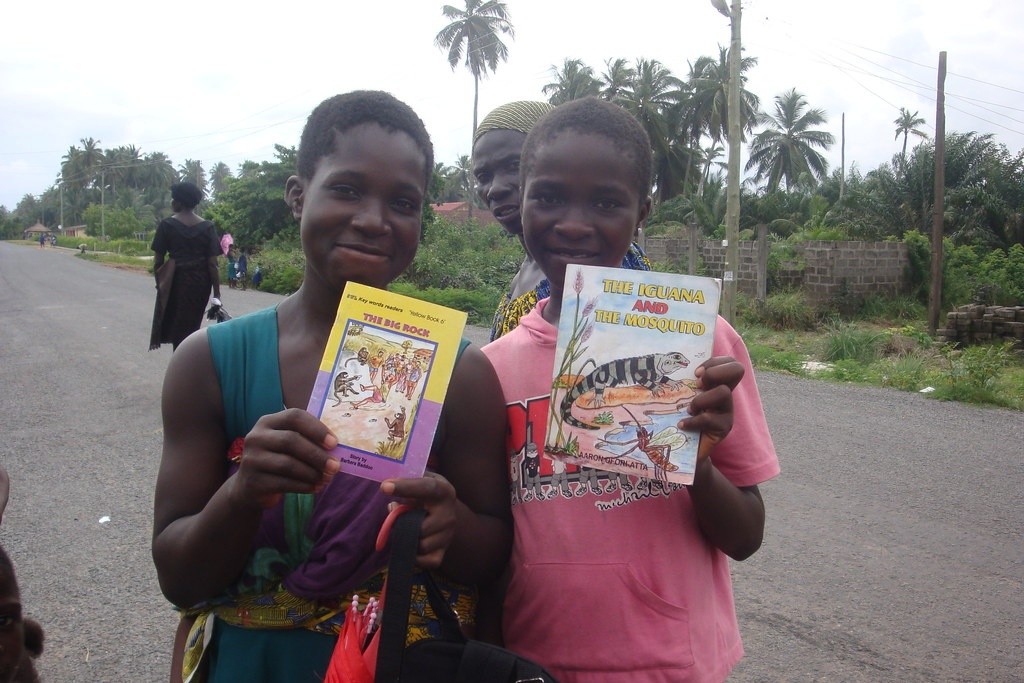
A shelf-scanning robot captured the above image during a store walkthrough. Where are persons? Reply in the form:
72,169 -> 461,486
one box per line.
40,233 -> 46,248
151,183 -> 224,354
227,244 -> 239,290
238,247 -> 248,290
151,89 -> 508,681
50,233 -> 57,248
482,98 -> 784,683
471,101 -> 655,342
253,263 -> 263,291
2,545 -> 25,681
76,243 -> 88,254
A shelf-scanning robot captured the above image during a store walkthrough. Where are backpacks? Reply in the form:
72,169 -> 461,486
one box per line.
375,514 -> 561,683
